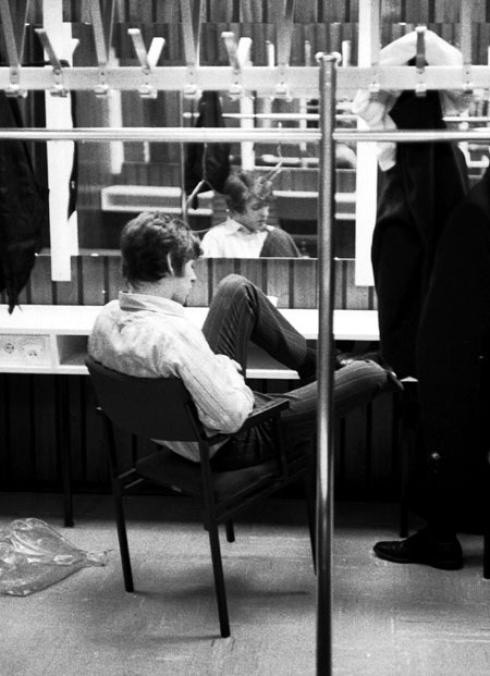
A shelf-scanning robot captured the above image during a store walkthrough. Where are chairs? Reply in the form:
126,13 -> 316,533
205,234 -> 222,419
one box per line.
81,350 -> 318,641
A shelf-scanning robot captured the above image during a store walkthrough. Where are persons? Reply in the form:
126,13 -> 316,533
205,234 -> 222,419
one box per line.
88,213 -> 422,469
198,168 -> 300,258
373,155 -> 490,569
355,26 -> 472,368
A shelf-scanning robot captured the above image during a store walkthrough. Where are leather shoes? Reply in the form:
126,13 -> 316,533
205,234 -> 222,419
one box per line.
482,542 -> 490,579
373,533 -> 467,570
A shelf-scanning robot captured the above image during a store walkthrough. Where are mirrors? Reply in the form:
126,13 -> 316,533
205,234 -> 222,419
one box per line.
196,15 -> 362,265
18,16 -> 190,263
377,15 -> 487,219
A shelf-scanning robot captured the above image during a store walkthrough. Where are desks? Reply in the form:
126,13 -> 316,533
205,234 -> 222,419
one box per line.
1,306 -> 379,527
